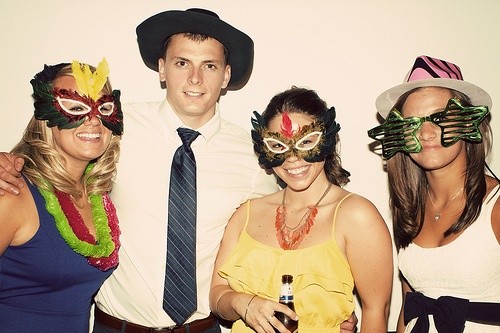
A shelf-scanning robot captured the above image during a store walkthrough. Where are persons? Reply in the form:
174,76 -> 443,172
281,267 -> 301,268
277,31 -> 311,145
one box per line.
368,56 -> 500,333
0,59 -> 124,333
0,7 -> 355,333
209,86 -> 394,333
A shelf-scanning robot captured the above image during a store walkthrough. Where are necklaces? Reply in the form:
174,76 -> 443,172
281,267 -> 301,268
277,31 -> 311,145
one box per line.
35,163 -> 121,270
276,183 -> 331,250
427,185 -> 463,221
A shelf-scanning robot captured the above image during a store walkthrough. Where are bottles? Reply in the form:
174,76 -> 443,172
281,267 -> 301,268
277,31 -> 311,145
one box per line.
272,275 -> 298,333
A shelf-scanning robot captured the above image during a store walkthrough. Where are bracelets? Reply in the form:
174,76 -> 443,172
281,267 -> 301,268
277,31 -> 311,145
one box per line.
215,290 -> 234,320
245,295 -> 257,321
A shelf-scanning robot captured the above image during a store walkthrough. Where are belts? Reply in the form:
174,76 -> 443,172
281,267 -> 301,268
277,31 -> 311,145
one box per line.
94,303 -> 219,333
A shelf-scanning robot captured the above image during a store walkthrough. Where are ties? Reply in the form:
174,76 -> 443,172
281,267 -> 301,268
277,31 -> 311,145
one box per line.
162,128 -> 201,327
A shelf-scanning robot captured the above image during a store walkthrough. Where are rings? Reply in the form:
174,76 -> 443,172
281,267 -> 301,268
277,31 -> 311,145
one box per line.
268,316 -> 273,321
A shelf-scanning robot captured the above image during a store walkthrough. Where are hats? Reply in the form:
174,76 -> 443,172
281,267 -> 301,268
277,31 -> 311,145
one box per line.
136,8 -> 254,90
375,55 -> 492,120
